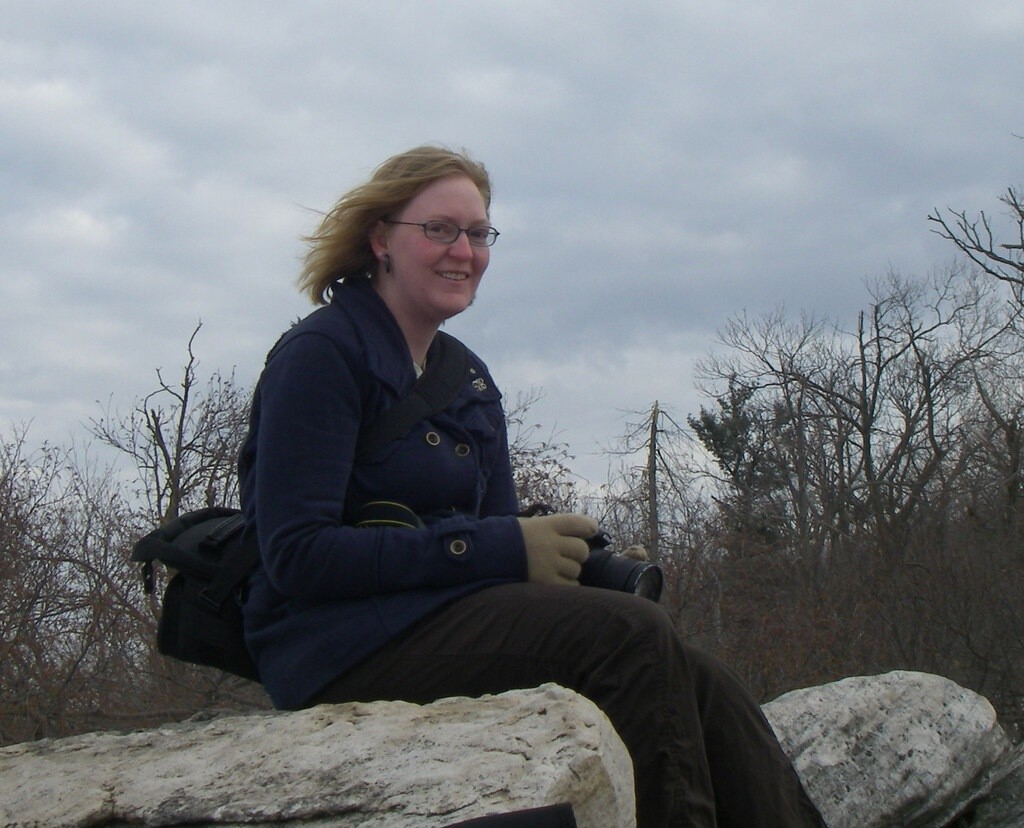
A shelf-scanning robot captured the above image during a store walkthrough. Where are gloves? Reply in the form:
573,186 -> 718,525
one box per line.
515,512 -> 599,586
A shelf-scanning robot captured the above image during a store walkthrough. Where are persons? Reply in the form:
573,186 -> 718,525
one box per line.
236,145 -> 829,828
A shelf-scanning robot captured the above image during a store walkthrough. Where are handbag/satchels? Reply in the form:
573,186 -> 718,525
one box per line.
129,507 -> 257,684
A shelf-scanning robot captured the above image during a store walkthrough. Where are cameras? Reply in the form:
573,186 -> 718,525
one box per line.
577,530 -> 662,604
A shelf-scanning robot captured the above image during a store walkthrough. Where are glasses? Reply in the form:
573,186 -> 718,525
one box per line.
380,219 -> 501,247
